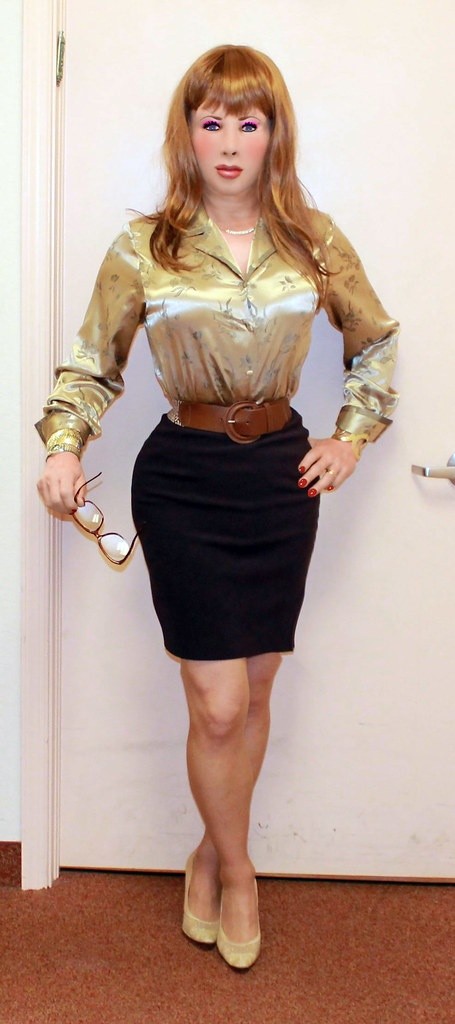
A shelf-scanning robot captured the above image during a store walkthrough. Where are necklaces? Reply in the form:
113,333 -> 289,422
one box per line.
210,225 -> 257,239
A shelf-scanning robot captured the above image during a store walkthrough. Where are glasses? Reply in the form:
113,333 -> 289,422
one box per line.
70,471 -> 138,565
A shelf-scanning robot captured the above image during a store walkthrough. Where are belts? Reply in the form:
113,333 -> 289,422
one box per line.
166,398 -> 292,445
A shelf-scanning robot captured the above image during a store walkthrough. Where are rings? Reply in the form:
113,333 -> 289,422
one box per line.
325,469 -> 337,480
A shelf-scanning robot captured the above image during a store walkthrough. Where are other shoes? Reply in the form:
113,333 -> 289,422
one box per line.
217,876 -> 262,970
182,848 -> 221,944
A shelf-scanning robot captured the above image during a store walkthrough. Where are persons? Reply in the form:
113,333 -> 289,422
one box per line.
35,47 -> 401,969
